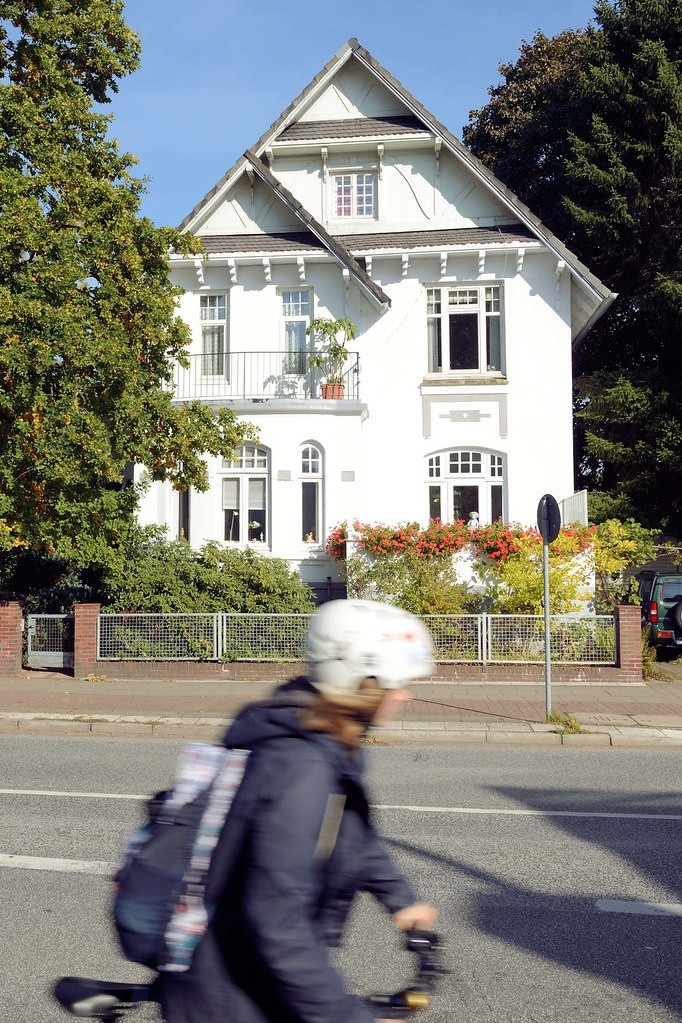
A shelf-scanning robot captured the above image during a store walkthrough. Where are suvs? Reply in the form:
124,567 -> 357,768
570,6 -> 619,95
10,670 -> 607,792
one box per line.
620,570 -> 682,646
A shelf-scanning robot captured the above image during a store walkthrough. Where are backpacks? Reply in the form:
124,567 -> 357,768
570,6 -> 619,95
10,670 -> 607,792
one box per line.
113,736 -> 347,975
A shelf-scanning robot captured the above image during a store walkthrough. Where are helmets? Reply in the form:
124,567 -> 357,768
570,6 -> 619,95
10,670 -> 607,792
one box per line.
304,598 -> 435,690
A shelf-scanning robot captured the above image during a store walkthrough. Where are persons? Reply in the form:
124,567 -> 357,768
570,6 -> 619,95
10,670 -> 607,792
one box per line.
466,511 -> 480,532
158,600 -> 439,1023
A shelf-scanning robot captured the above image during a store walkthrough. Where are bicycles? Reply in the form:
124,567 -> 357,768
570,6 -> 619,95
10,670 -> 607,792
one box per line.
54,927 -> 441,1023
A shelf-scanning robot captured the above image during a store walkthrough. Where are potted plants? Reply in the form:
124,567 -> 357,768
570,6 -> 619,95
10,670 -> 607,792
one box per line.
304,314 -> 359,400
